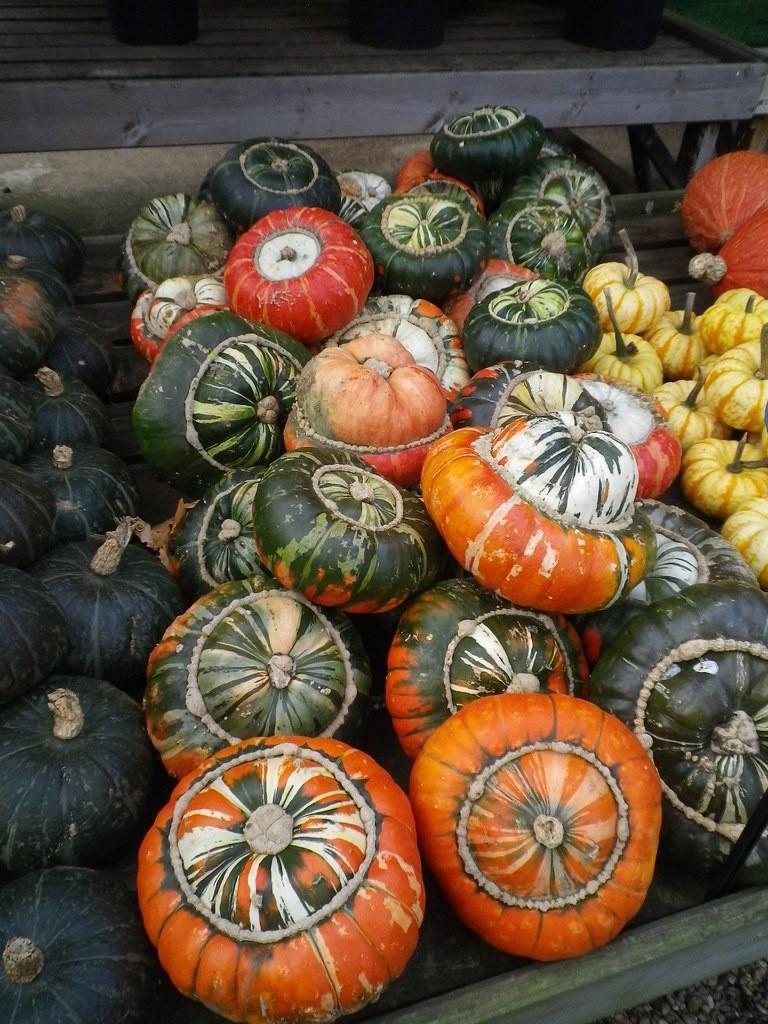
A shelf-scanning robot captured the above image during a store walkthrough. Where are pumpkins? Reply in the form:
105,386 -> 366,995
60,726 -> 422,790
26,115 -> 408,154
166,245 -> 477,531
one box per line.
0,105 -> 768,1024
